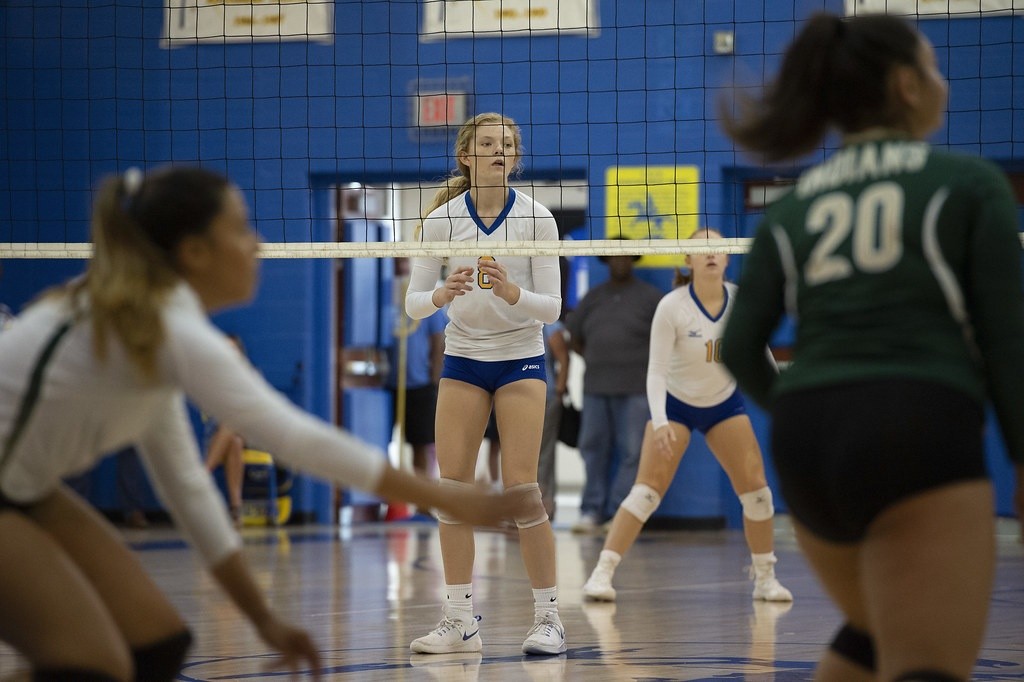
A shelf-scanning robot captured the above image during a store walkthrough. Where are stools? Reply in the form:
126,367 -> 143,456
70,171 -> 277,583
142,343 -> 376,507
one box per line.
242,449 -> 275,528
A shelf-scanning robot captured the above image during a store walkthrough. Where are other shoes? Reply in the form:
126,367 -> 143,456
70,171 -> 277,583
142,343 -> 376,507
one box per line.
596,520 -> 613,539
571,516 -> 595,532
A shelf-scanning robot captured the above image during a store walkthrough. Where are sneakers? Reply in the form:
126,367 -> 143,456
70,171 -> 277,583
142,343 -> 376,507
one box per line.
409,607 -> 483,654
582,603 -> 616,630
522,653 -> 566,682
752,578 -> 792,602
410,651 -> 482,682
521,609 -> 566,653
582,568 -> 617,603
752,602 -> 793,620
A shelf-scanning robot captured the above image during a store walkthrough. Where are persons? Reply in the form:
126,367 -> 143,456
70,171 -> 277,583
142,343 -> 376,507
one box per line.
719,12 -> 1024,682
571,235 -> 666,532
484,321 -> 569,522
190,333 -> 244,525
391,254 -> 449,517
0,164 -> 537,682
405,114 -> 566,654
583,229 -> 794,601
